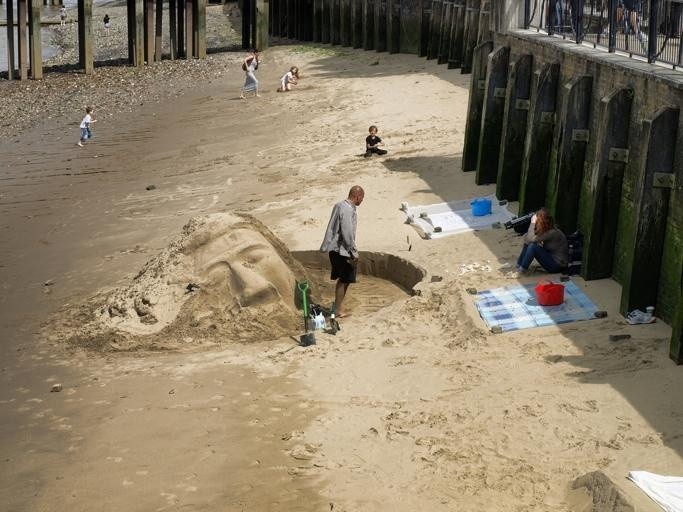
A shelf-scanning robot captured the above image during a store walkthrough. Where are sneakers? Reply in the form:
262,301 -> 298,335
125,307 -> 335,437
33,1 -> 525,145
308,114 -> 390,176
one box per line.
628,313 -> 657,325
625,309 -> 653,322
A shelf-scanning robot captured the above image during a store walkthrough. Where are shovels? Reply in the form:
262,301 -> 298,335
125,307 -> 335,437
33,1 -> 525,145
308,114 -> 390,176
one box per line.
298,280 -> 316,347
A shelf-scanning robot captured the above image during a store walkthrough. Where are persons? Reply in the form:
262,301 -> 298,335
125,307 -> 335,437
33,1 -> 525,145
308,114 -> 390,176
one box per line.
600,0 -> 647,41
59,5 -> 67,25
666,1 -> 682,39
621,0 -> 641,35
277,65 -> 298,91
179,211 -> 313,320
319,185 -> 364,317
504,206 -> 569,279
364,126 -> 387,156
102,14 -> 110,37
239,48 -> 260,99
77,107 -> 98,146
